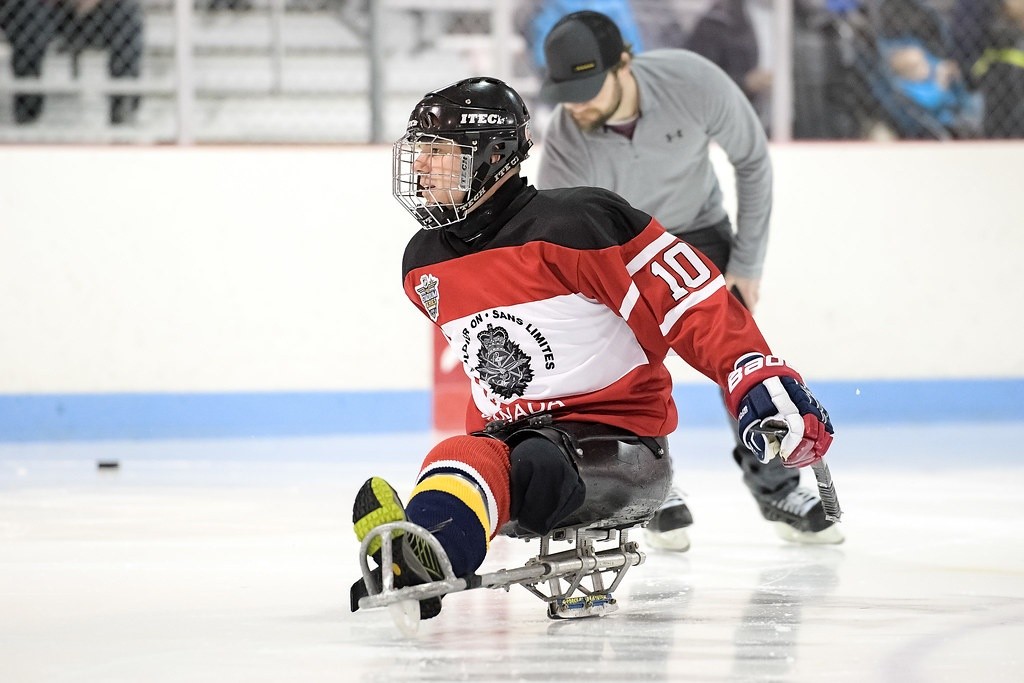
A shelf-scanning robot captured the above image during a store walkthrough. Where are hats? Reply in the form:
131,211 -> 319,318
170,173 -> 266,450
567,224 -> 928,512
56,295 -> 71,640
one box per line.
540,10 -> 623,103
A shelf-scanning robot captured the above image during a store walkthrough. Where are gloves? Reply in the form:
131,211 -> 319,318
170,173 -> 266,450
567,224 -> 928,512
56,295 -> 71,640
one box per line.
737,376 -> 834,469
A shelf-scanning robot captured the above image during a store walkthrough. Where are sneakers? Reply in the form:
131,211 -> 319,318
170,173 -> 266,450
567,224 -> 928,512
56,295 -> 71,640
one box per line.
641,487 -> 695,556
352,476 -> 444,618
754,489 -> 845,544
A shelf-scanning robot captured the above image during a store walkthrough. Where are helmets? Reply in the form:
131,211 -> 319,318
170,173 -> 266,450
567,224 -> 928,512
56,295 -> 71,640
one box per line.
392,77 -> 535,232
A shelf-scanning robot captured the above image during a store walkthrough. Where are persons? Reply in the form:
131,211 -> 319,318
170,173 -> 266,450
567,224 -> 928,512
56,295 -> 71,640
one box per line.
348,76 -> 838,634
537,10 -> 847,553
685,0 -> 1024,142
1,0 -> 145,126
